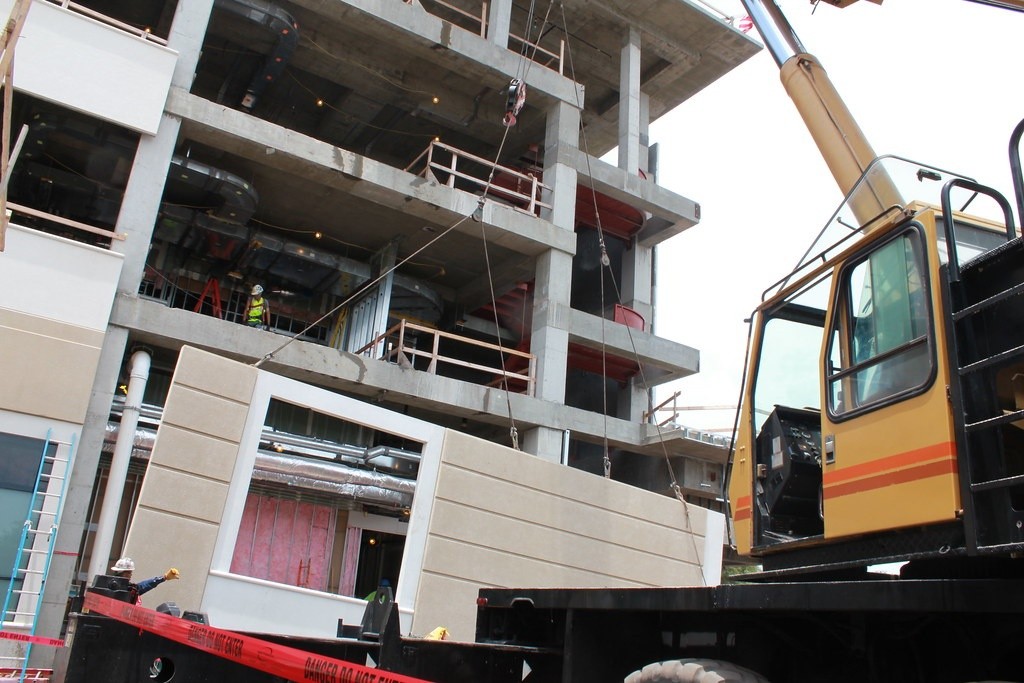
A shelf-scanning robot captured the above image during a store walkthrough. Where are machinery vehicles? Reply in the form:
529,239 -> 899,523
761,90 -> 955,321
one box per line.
717,0 -> 1024,581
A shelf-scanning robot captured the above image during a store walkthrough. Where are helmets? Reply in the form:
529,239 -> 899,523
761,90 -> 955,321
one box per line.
251,284 -> 263,295
110,557 -> 135,574
379,578 -> 390,587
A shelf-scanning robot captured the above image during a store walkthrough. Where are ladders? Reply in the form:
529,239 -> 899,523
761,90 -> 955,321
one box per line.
2,429 -> 80,683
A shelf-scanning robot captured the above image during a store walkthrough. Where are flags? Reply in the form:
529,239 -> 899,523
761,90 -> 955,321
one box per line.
737,14 -> 753,35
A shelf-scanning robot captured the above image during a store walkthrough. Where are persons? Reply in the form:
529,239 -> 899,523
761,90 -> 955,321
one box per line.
111,557 -> 181,605
243,284 -> 272,332
364,579 -> 390,604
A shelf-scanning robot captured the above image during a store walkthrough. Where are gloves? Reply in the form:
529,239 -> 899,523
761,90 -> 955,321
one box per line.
265,325 -> 270,331
242,320 -> 246,325
163,568 -> 180,580
